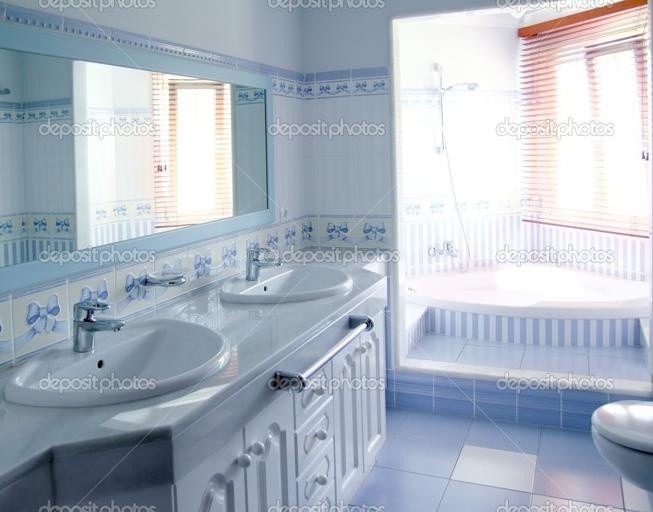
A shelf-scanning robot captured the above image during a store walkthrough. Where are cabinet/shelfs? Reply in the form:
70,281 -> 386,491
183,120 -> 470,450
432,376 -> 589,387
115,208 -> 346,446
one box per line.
294,338 -> 337,510
165,363 -> 299,512
332,307 -> 388,512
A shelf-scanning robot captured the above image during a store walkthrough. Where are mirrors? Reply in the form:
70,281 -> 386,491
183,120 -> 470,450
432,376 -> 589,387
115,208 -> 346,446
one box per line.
2,1 -> 275,300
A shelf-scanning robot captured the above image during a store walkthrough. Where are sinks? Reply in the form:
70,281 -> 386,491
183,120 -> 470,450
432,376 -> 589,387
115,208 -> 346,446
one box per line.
4,316 -> 232,408
219,262 -> 354,304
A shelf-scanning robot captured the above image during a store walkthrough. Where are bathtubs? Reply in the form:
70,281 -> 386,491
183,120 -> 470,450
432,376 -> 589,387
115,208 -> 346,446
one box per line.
405,263 -> 652,321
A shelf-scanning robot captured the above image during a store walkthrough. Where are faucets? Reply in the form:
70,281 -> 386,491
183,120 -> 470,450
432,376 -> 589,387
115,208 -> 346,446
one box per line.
257,261 -> 283,269
82,316 -> 125,332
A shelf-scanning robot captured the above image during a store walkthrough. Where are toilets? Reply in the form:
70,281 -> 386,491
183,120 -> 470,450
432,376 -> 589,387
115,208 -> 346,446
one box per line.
589,399 -> 653,511
